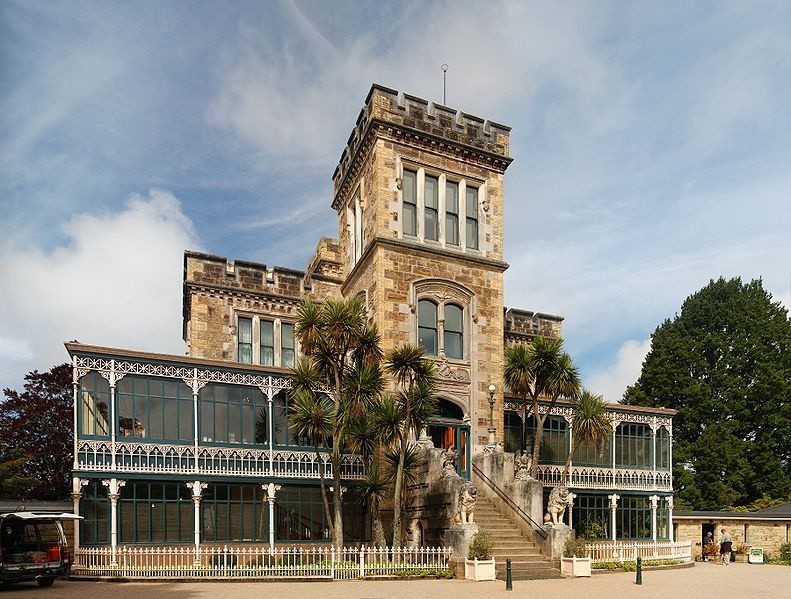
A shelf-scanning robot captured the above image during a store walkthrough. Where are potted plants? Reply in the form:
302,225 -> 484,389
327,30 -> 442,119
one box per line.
561,535 -> 591,578
465,528 -> 497,581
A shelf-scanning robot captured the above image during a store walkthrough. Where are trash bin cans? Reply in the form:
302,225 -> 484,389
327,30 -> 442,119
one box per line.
748,548 -> 763,564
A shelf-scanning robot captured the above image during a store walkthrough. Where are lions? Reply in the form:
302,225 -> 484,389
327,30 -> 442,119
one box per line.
544,484 -> 569,525
454,480 -> 477,523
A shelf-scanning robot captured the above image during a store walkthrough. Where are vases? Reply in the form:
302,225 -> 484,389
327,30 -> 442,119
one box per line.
707,555 -> 721,562
735,554 -> 749,562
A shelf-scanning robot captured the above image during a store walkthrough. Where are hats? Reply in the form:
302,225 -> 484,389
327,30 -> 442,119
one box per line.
708,532 -> 712,536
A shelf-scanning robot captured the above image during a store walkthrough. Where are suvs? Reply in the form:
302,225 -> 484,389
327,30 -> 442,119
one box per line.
0,511 -> 85,588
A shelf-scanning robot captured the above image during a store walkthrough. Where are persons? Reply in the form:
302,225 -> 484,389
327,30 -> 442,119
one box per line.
704,529 -> 732,566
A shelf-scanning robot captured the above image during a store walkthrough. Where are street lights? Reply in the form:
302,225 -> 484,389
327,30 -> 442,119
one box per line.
486,384 -> 500,450
